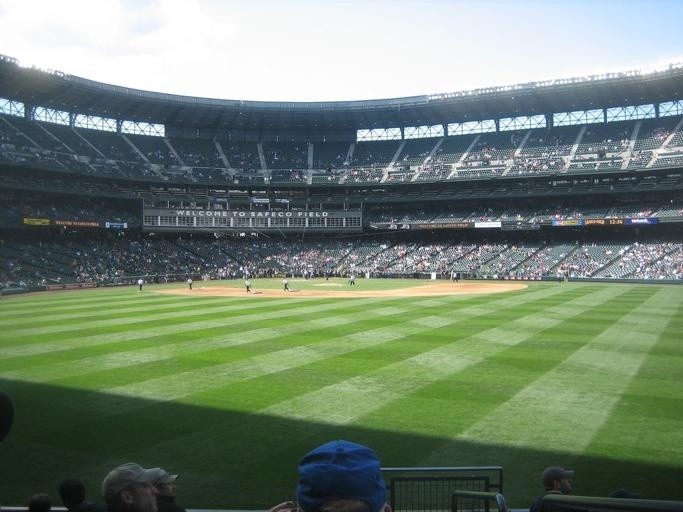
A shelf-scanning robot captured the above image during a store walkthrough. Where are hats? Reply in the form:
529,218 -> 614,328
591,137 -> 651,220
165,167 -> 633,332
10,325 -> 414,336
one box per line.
296,439 -> 387,511
541,466 -> 576,480
99,462 -> 163,500
143,468 -> 182,484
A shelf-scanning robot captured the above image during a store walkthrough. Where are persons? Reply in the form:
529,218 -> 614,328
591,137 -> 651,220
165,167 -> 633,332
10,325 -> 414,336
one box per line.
0,392 -> 15,440
607,489 -> 641,499
270,439 -> 393,512
529,466 -> 575,511
28,439 -> 186,510
